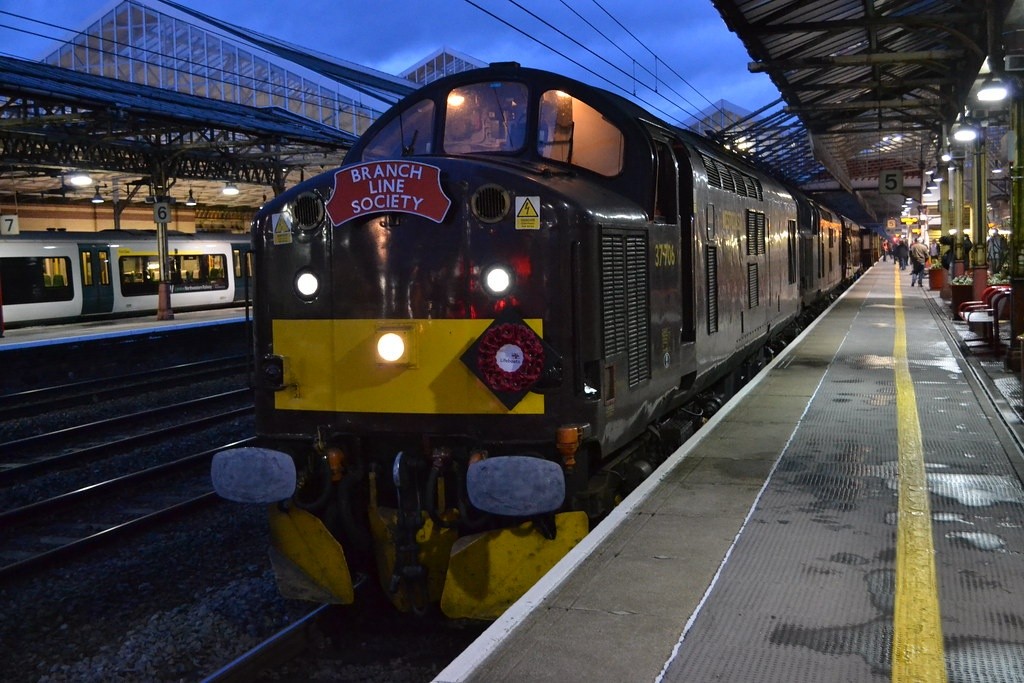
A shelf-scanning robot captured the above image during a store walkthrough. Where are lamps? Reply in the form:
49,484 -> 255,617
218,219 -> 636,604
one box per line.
977,75 -> 1007,102
954,123 -> 977,141
223,182 -> 240,195
92,189 -> 105,203
186,184 -> 196,204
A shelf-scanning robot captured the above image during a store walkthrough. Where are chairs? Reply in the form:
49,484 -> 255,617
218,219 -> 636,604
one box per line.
43,274 -> 64,286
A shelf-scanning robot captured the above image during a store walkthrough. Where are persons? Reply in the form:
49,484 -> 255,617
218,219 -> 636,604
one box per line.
882,236 -> 940,287
987,228 -> 1008,274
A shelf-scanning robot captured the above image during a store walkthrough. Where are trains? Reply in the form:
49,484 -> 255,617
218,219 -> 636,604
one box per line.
0,228 -> 256,328
211,61 -> 890,624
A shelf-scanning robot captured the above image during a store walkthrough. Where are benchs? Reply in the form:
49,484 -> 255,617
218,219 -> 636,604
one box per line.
958,284 -> 1013,355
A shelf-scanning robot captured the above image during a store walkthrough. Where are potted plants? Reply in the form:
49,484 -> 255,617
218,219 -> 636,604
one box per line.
928,263 -> 942,290
950,275 -> 974,319
